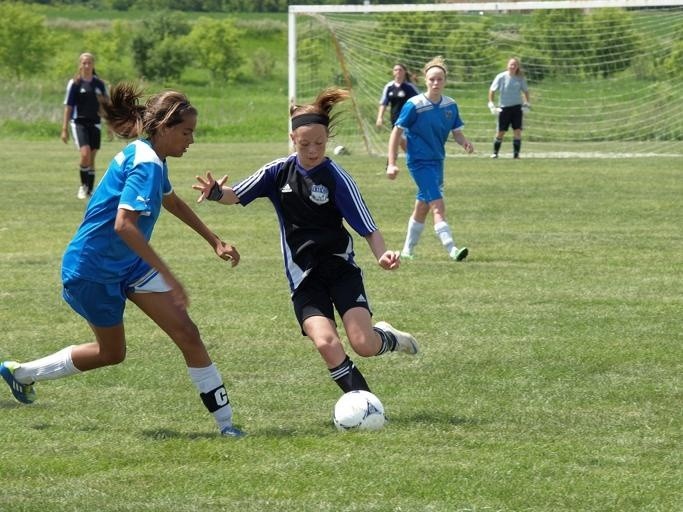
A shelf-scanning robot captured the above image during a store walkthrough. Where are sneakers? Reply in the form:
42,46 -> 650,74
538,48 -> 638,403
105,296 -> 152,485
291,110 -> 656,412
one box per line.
0,360 -> 36,404
77,184 -> 93,199
451,247 -> 469,261
221,426 -> 248,437
374,321 -> 419,355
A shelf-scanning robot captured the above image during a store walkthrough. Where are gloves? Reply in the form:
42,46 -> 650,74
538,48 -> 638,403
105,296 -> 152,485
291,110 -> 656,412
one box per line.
488,101 -> 503,113
521,101 -> 532,113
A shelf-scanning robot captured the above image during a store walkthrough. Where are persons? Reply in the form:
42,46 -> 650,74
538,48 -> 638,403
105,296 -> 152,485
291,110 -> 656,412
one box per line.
387,55 -> 475,262
377,63 -> 419,154
58,53 -> 111,201
0,80 -> 247,438
190,87 -> 420,395
485,56 -> 534,160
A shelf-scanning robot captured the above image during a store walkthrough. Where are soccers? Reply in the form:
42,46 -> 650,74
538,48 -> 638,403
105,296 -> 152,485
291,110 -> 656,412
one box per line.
333,390 -> 384,434
334,146 -> 348,155
